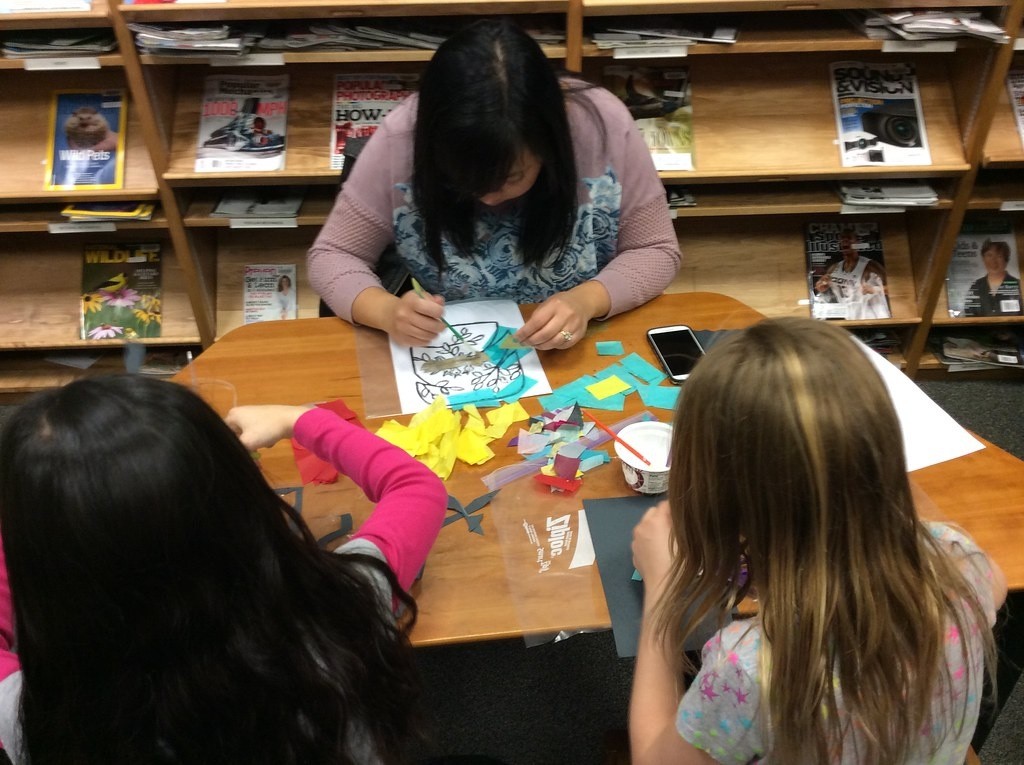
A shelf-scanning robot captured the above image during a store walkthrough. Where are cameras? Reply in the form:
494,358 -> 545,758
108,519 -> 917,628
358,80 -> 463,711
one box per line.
862,111 -> 924,147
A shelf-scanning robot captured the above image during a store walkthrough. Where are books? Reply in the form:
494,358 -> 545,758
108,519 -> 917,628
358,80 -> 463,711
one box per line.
932,328 -> 1023,372
124,13 -> 567,59
44,86 -> 127,195
591,19 -> 741,61
850,7 -> 1008,42
80,240 -> 163,341
829,60 -> 932,169
206,190 -> 305,220
578,57 -> 693,169
944,233 -> 1024,318
840,180 -> 937,207
63,200 -> 154,223
195,69 -> 289,174
1,30 -> 115,60
805,212 -> 893,322
329,72 -> 420,175
847,328 -> 900,354
242,264 -> 297,325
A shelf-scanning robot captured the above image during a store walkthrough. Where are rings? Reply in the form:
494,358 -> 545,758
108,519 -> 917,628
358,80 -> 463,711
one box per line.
561,330 -> 572,342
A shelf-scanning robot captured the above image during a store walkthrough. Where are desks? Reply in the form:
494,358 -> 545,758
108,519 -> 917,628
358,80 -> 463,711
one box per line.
178,289 -> 1024,756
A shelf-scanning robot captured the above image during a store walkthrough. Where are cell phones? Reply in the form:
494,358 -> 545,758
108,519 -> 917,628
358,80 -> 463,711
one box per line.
646,324 -> 706,383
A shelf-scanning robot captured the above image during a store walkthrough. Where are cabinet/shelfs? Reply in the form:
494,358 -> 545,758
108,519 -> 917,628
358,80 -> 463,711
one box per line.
0,1 -> 1024,381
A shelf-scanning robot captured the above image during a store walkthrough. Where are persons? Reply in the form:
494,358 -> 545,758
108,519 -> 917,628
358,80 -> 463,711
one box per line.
307,18 -> 683,352
0,374 -> 449,765
814,230 -> 888,302
964,240 -> 1021,315
274,274 -> 295,319
628,315 -> 997,765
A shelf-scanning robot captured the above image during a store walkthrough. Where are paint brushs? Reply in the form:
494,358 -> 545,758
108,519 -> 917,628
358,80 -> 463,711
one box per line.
410,277 -> 464,342
579,409 -> 652,466
